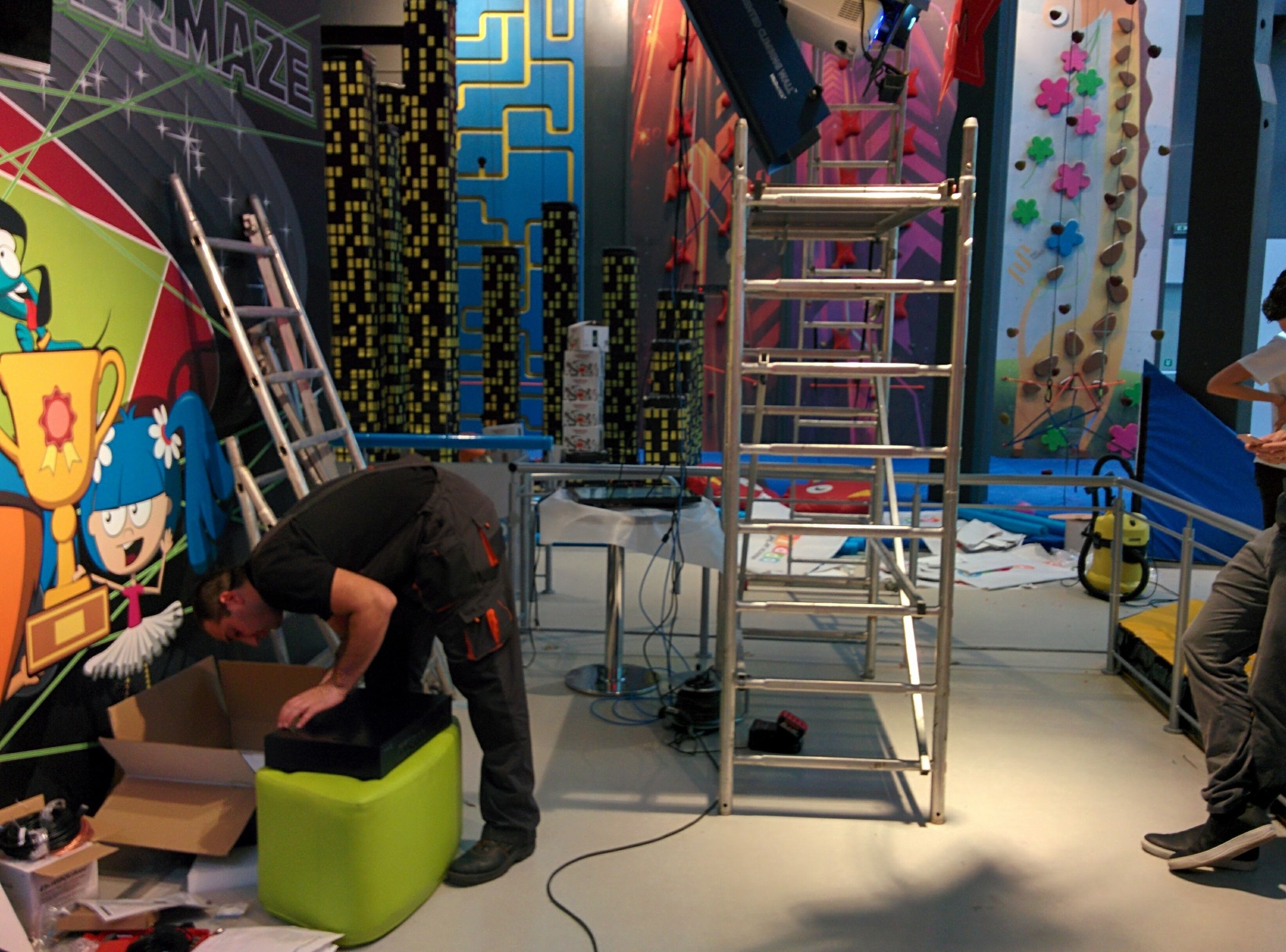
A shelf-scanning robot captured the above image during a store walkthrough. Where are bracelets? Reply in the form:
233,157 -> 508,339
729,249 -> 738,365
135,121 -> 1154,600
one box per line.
1276,394 -> 1286,408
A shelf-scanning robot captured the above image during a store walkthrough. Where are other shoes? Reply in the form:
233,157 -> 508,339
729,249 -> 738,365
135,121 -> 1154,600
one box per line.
1142,819 -> 1260,871
1168,797 -> 1277,869
448,823 -> 537,886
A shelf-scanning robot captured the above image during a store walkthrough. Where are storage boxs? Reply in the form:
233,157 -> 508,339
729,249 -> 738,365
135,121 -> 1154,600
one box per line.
558,319 -> 610,454
89,654 -> 328,857
0,793 -> 119,944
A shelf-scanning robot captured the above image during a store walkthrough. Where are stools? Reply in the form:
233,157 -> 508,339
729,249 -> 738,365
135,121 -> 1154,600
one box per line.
252,687 -> 464,946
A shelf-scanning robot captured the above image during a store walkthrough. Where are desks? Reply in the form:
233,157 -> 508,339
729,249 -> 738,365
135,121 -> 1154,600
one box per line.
537,486 -> 728,699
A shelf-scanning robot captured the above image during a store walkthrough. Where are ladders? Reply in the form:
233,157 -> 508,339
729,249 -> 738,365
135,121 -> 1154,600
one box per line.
170,170 -> 460,703
786,39 -> 913,574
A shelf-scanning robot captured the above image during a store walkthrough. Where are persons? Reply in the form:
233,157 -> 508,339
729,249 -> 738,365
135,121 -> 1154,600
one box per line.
1207,329 -> 1286,529
1142,271 -> 1286,869
193,456 -> 543,890
